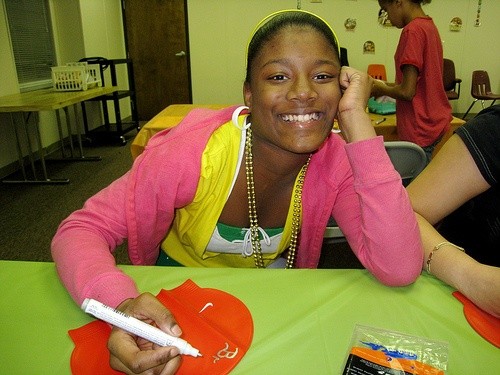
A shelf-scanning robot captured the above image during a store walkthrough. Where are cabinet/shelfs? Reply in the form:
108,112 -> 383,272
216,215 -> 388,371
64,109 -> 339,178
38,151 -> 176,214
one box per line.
79,59 -> 139,148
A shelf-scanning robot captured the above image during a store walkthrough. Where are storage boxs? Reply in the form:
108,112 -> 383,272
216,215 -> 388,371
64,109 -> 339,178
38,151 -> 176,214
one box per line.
50,62 -> 103,92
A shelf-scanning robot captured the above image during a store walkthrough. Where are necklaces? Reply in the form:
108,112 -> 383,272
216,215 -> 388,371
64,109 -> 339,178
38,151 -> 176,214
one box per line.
245,118 -> 312,269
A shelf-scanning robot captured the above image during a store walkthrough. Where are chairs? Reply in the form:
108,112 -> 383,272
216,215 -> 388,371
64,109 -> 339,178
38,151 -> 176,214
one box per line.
323,140 -> 428,267
461,71 -> 500,119
367,64 -> 386,81
443,58 -> 462,100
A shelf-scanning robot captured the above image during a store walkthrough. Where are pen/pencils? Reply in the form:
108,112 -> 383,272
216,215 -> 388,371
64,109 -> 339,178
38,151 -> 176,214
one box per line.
376,117 -> 386,124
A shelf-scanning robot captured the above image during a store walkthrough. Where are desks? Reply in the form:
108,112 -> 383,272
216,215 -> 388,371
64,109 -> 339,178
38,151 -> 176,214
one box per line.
131,102 -> 466,166
0,261 -> 500,375
0,87 -> 122,183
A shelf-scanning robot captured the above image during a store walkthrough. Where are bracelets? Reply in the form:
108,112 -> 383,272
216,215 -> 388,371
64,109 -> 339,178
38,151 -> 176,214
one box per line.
427,242 -> 464,273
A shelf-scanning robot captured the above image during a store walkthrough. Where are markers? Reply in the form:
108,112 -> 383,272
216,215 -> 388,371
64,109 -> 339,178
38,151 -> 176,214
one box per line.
80,298 -> 203,357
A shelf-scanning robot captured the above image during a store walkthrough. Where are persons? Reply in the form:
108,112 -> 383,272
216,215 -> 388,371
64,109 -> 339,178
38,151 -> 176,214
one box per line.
407,103 -> 500,319
365,0 -> 453,181
51,10 -> 424,375
